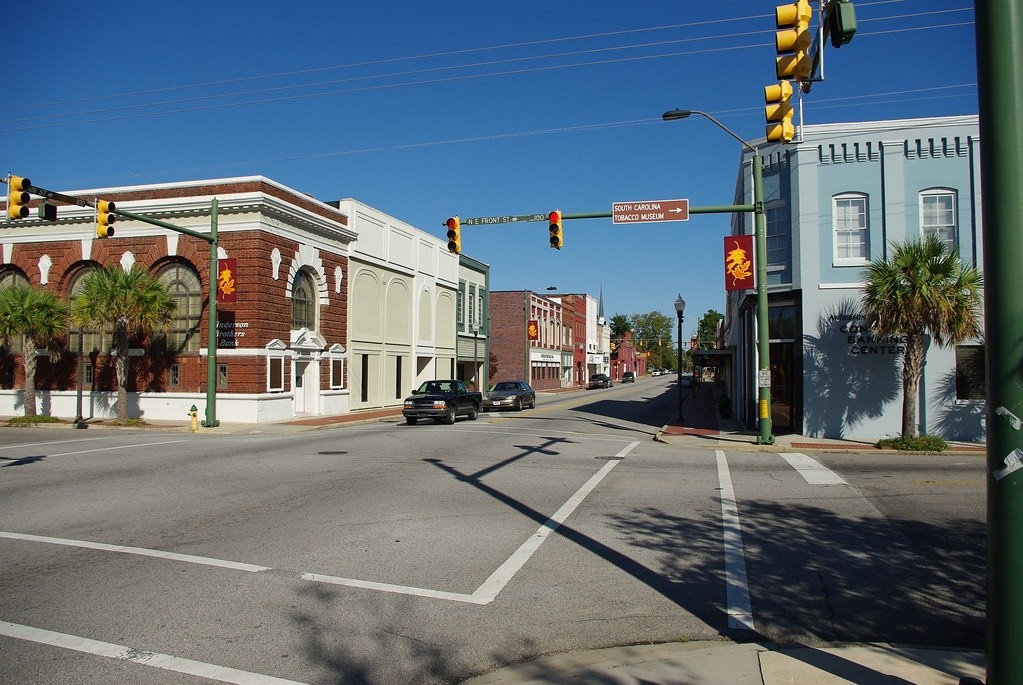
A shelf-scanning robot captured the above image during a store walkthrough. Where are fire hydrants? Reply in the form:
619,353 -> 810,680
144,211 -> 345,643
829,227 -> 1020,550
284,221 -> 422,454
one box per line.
187,405 -> 198,432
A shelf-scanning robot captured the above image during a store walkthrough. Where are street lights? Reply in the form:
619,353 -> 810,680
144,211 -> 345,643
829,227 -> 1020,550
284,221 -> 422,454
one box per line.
472,320 -> 479,393
660,108 -> 775,444
524,286 -> 557,382
73,281 -> 86,424
674,293 -> 685,425
691,328 -> 698,400
579,344 -> 583,389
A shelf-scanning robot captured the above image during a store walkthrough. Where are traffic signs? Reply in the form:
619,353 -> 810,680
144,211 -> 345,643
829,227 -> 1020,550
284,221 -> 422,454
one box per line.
612,199 -> 690,224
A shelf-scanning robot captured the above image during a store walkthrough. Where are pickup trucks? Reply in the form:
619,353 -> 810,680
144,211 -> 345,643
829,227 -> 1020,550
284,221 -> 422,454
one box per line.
402,379 -> 482,424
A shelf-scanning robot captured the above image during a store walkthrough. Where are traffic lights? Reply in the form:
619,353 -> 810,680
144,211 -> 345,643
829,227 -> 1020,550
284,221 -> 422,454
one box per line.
611,343 -> 616,351
96,199 -> 115,238
667,342 -> 669,349
548,209 -> 563,248
446,216 -> 461,253
775,0 -> 813,82
764,79 -> 795,145
8,173 -> 31,219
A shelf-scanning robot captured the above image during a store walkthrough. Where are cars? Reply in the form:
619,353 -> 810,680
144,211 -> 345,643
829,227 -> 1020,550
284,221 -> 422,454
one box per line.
651,368 -> 678,377
622,371 -> 634,383
481,379 -> 535,413
589,374 -> 613,389
